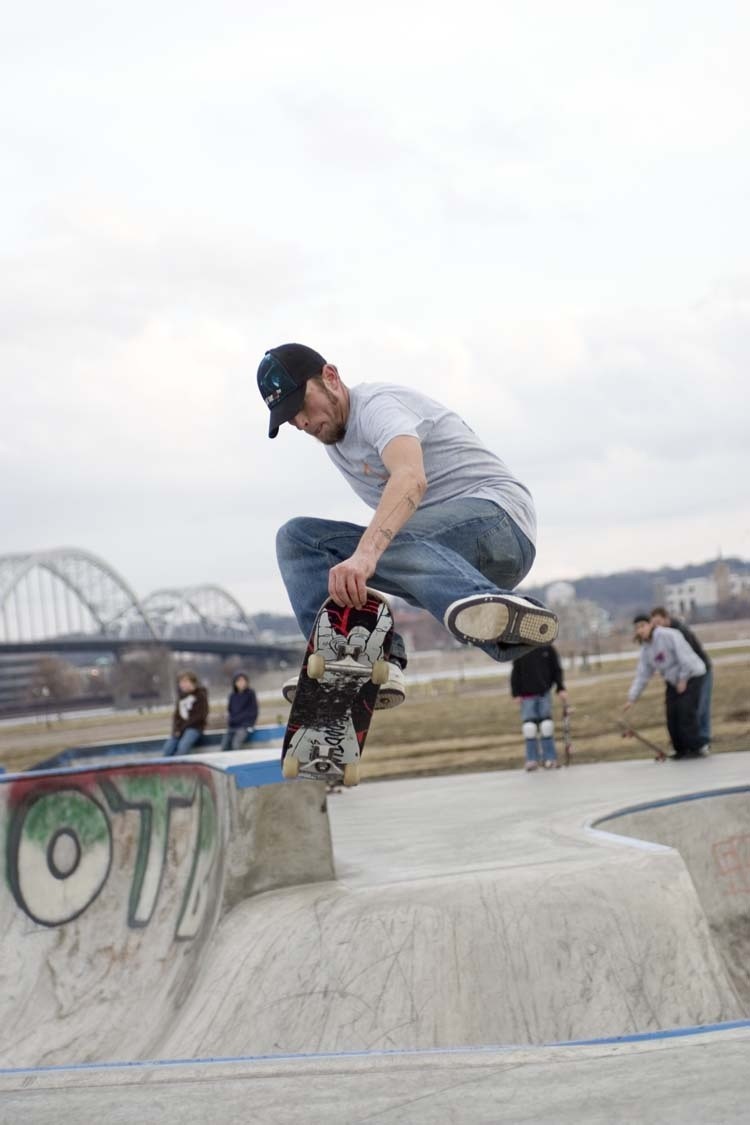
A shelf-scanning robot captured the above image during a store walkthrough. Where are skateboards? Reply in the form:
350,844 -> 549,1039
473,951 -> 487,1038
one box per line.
563,702 -> 578,766
608,711 -> 672,762
280,587 -> 394,786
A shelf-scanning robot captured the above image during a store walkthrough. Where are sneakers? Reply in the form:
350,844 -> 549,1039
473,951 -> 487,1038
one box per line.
442,594 -> 559,647
284,661 -> 405,711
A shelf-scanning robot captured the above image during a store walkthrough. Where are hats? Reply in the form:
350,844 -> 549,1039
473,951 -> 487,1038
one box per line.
257,344 -> 326,439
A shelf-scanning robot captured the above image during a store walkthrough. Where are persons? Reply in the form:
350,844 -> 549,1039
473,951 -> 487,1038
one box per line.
161,670 -> 209,756
651,606 -> 714,757
508,640 -> 569,771
255,345 -> 559,711
623,613 -> 710,758
218,672 -> 258,751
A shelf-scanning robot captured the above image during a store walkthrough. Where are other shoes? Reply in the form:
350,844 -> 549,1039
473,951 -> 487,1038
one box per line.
544,760 -> 559,768
526,761 -> 537,771
